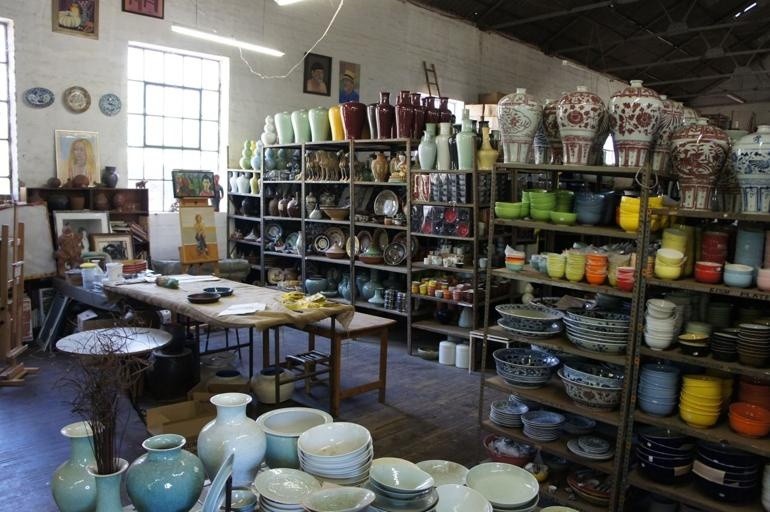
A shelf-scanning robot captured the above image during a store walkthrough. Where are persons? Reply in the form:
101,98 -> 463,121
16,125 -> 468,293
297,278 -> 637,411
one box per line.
105,241 -> 128,260
195,213 -> 211,256
339,69 -> 358,103
175,173 -> 196,196
306,60 -> 328,94
60,138 -> 98,186
209,173 -> 223,212
78,228 -> 93,254
198,176 -> 214,196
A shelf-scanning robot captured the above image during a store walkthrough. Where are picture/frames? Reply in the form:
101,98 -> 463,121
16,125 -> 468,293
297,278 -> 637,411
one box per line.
54,130 -> 100,187
37,293 -> 68,354
90,232 -> 135,264
52,0 -> 99,40
39,288 -> 55,322
122,0 -> 164,19
303,53 -> 332,96
53,210 -> 110,278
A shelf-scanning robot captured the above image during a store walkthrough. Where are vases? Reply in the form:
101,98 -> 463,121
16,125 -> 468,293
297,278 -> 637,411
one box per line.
417,119 -> 498,170
498,79 -> 707,175
262,91 -> 455,145
51,393 -> 266,512
230,139 -> 300,193
671,116 -> 770,214
104,167 -> 117,187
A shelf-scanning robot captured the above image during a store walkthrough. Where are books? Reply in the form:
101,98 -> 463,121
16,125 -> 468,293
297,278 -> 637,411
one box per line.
108,214 -> 151,243
7,288 -> 39,340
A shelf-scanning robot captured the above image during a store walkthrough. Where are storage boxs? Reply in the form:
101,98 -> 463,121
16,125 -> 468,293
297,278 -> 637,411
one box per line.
77,310 -> 112,331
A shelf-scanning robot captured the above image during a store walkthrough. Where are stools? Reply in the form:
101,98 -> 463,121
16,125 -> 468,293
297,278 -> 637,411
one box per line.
262,311 -> 397,415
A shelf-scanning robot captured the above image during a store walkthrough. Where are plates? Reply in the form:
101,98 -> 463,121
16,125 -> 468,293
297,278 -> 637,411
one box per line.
203,287 -> 233,296
373,190 -> 399,218
297,422 -> 374,484
301,486 -> 377,512
267,267 -> 285,285
266,224 -> 418,266
421,222 -> 432,233
368,490 -> 440,512
444,208 -> 457,224
202,453 -> 234,512
98,93 -> 122,116
455,222 -> 470,237
415,460 -> 469,485
187,293 -> 221,303
433,483 -> 495,512
369,456 -> 436,504
23,88 -> 55,108
485,394 -> 616,509
464,462 -> 540,512
255,469 -> 321,512
62,86 -> 93,114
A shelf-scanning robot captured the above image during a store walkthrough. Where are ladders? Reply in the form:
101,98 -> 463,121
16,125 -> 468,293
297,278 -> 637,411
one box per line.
422,60 -> 441,99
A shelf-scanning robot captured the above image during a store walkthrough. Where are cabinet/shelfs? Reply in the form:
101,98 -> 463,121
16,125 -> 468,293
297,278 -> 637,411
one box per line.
20,187 -> 151,270
227,169 -> 261,285
479,161 -> 769,512
261,138 -> 559,370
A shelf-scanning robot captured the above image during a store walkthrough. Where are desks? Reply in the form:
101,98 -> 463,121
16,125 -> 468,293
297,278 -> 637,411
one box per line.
55,326 -> 171,357
103,275 -> 355,419
53,277 -> 118,310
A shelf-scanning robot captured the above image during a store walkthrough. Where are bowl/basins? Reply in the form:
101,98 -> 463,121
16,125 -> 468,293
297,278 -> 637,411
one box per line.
489,291 -> 628,413
64,268 -> 82,286
319,207 -> 350,221
417,346 -> 440,360
635,219 -> 770,436
505,248 -> 653,291
495,186 -> 667,233
220,488 -> 258,512
631,431 -> 770,512
257,406 -> 332,468
123,260 -> 146,274
412,275 -> 507,301
199,350 -> 238,368
320,290 -> 337,298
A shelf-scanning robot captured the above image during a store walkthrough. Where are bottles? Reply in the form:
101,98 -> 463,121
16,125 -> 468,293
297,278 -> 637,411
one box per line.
197,392 -> 267,489
63,311 -> 156,512
51,420 -> 106,512
125,434 -> 203,512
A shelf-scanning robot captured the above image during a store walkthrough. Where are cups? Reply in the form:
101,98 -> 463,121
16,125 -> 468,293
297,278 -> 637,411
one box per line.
383,216 -> 393,226
106,262 -> 124,279
394,217 -> 404,226
80,263 -> 98,289
421,245 -> 488,270
437,309 -> 453,324
479,222 -> 486,237
